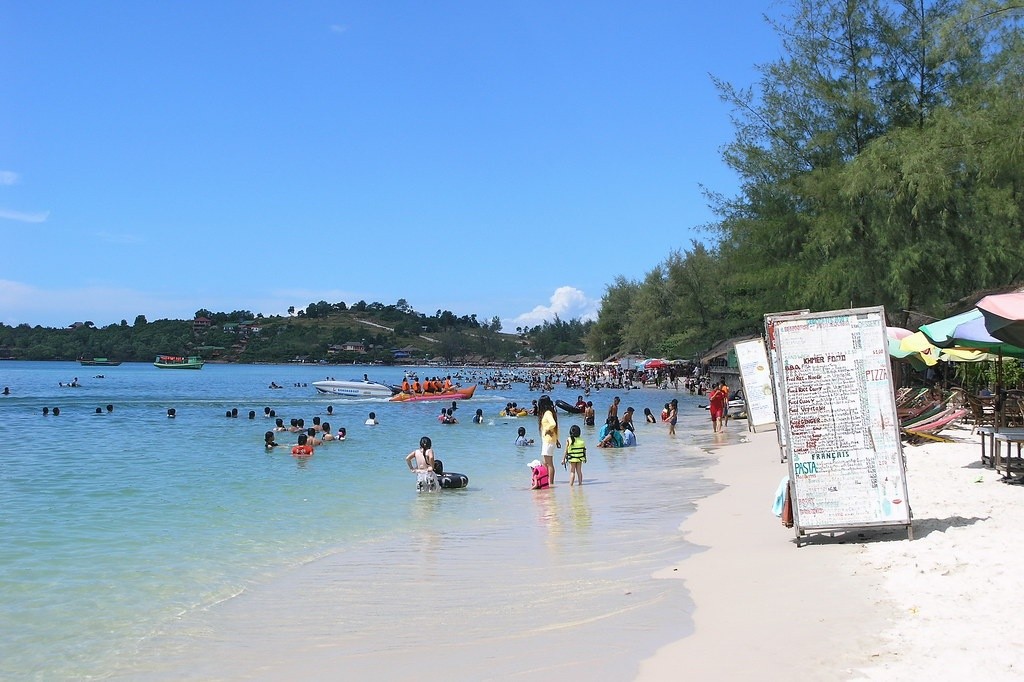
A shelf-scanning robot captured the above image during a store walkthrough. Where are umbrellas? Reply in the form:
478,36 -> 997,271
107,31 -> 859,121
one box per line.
225,365 -> 729,494
640,358 -> 666,367
662,358 -> 691,365
884,290 -> 1024,428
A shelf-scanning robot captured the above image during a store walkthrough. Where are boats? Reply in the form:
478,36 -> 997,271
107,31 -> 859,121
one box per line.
78,357 -> 123,366
152,354 -> 205,369
389,384 -> 477,401
311,381 -> 395,397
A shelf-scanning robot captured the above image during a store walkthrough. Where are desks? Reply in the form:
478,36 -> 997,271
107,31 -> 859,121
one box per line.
977,426 -> 1024,477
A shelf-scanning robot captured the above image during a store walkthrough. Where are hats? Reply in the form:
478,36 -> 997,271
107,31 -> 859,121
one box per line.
527,459 -> 541,469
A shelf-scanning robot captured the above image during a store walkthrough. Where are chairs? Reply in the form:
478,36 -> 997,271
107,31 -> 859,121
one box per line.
895,385 -> 1024,443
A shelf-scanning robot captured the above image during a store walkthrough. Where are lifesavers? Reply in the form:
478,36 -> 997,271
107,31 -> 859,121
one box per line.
500,409 -> 528,416
556,399 -> 580,413
432,471 -> 468,488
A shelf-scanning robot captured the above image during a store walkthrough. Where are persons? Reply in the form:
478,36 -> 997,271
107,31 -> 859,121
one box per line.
442,409 -> 459,424
161,356 -> 187,363
264,431 -> 279,451
527,460 -> 549,490
364,412 -> 378,425
326,406 -> 334,415
514,427 -> 534,446
473,409 -> 486,424
1,374 -> 176,419
406,437 -> 442,493
897,392 -> 943,415
584,401 -> 595,426
643,408 -> 656,423
247,411 -> 256,419
105,404 -> 115,412
561,424 -> 587,486
2,387 -> 12,395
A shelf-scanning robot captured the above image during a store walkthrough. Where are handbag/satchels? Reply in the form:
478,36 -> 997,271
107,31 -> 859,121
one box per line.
782,481 -> 794,528
772,475 -> 789,518
